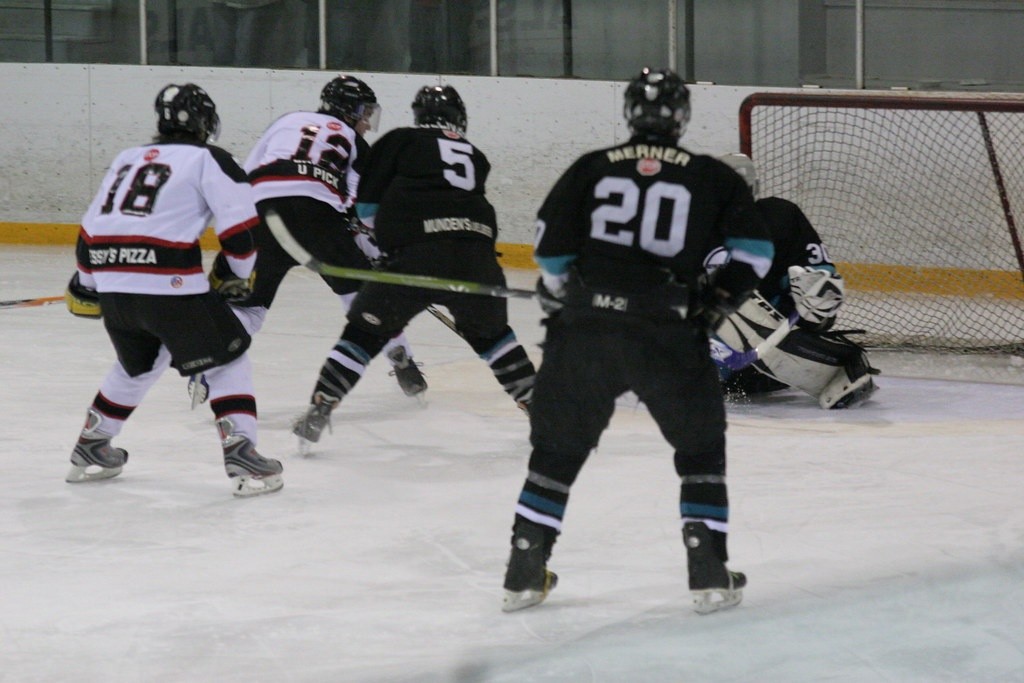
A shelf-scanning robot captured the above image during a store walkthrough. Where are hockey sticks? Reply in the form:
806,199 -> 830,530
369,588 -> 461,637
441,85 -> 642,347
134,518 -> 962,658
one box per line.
708,313 -> 802,372
426,304 -> 545,353
0,296 -> 65,309
264,209 -> 536,301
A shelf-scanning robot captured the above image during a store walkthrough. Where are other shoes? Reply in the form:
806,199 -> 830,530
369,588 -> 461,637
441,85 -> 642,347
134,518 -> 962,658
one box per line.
833,381 -> 881,410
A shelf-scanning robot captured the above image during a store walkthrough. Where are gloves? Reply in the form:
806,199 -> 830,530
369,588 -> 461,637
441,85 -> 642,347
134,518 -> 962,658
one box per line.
536,274 -> 565,315
704,263 -> 748,337
65,269 -> 104,319
209,263 -> 253,304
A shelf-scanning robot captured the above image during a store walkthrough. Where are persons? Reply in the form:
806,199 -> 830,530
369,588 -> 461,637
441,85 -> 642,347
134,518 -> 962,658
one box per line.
61,83 -> 287,500
288,81 -> 537,453
705,150 -> 881,409
496,67 -> 773,620
301,0 -> 377,70
404,0 -> 476,75
184,74 -> 431,408
210,0 -> 285,67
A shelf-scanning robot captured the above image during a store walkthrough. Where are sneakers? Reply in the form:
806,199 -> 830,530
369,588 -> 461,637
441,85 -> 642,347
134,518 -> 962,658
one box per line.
387,344 -> 430,408
502,522 -> 559,613
214,418 -> 283,497
187,369 -> 210,409
517,387 -> 535,418
65,408 -> 128,483
291,390 -> 341,457
682,522 -> 746,615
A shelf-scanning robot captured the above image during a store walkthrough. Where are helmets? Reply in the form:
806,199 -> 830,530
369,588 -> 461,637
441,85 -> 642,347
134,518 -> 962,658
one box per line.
716,152 -> 759,197
412,85 -> 469,132
155,83 -> 215,143
624,66 -> 691,139
320,73 -> 377,120
